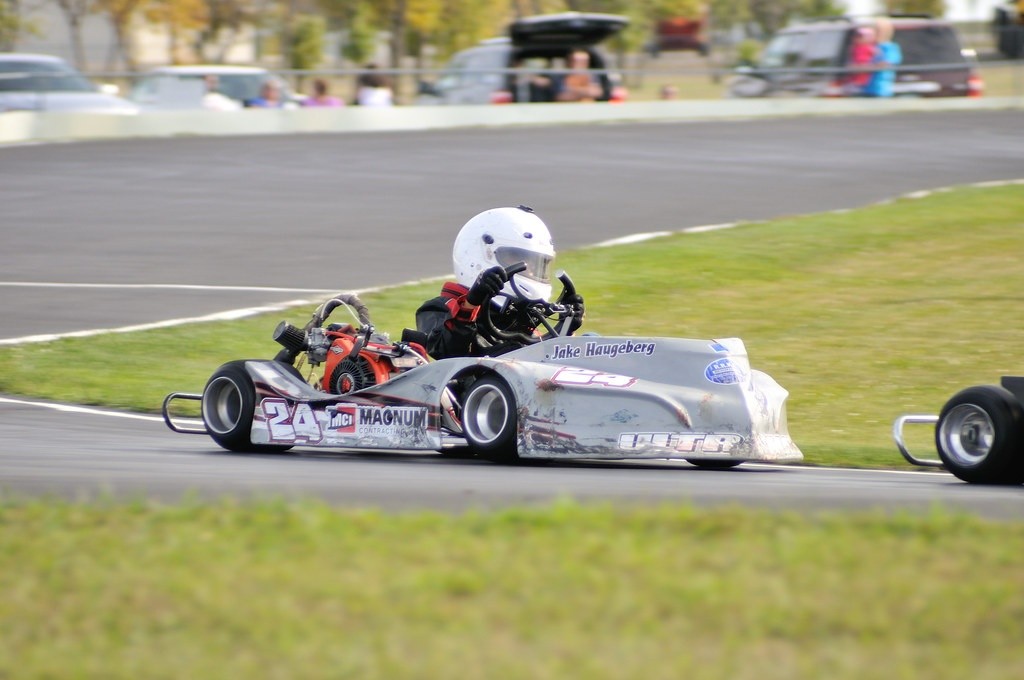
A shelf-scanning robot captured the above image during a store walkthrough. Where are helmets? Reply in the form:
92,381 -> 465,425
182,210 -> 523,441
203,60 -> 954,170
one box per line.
452,204 -> 555,308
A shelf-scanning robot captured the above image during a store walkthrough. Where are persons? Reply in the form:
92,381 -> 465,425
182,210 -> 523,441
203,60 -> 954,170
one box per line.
303,79 -> 342,107
201,74 -> 222,91
850,20 -> 901,96
249,79 -> 286,108
358,65 -> 392,106
415,204 -> 585,360
510,49 -> 603,102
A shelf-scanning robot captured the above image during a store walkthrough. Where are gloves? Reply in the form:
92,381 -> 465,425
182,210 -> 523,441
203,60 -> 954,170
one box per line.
466,265 -> 507,306
558,294 -> 585,336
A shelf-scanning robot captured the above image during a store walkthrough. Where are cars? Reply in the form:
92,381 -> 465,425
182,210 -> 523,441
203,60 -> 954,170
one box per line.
134,63 -> 312,112
0,52 -> 143,114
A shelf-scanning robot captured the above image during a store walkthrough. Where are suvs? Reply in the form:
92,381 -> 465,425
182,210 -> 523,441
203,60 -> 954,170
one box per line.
416,12 -> 632,104
729,13 -> 986,102
